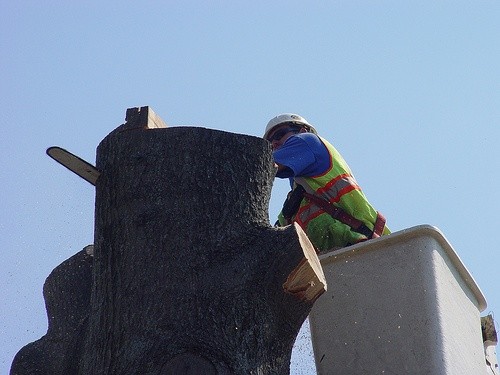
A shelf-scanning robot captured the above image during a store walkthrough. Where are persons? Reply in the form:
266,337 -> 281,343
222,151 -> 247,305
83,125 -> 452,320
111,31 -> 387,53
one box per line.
263,113 -> 393,254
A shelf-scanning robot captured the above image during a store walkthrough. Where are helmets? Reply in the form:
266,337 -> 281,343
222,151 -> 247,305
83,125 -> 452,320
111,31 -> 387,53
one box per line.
262,114 -> 318,138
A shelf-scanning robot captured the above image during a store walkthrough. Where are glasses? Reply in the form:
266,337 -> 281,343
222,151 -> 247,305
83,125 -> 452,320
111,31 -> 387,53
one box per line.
268,126 -> 299,143
